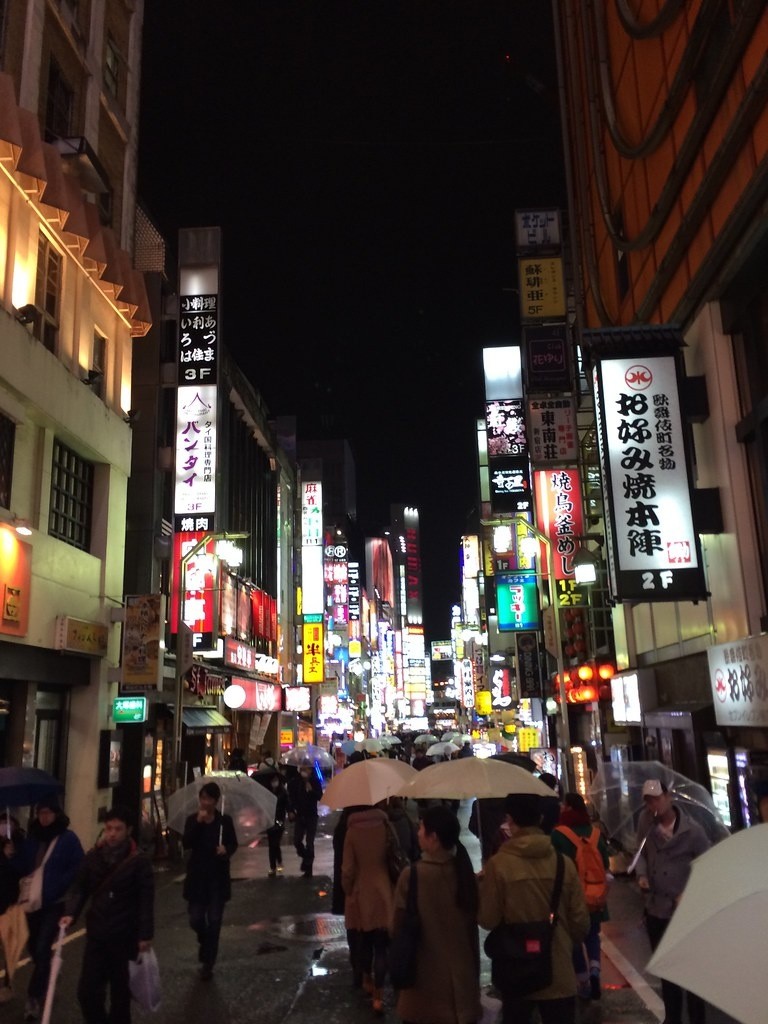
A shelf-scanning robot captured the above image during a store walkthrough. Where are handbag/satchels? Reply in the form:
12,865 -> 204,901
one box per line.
16,867 -> 42,912
128,947 -> 164,1013
384,909 -> 422,990
484,920 -> 556,996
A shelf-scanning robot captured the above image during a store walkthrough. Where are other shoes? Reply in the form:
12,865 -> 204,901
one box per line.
361,969 -> 375,992
372,987 -> 385,1010
305,870 -> 313,879
299,857 -> 305,871
577,967 -> 601,1002
268,865 -> 283,875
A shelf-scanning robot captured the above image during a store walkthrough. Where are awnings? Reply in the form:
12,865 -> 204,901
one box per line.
168,708 -> 233,735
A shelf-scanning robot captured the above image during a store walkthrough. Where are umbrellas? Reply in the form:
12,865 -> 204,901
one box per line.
0,766 -> 64,839
167,770 -> 278,855
41,923 -> 67,1024
279,742 -> 337,767
395,752 -> 559,869
641,821 -> 768,1024
360,732 -> 472,755
585,761 -> 732,874
0,901 -> 29,990
319,751 -> 419,811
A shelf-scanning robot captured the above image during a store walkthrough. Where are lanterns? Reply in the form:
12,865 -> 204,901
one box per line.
472,691 -> 538,752
553,662 -> 613,705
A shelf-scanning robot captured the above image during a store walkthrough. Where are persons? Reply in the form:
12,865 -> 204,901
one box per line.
59,805 -> 154,1024
182,781 -> 239,980
636,780 -> 712,1024
228,749 -> 278,774
349,735 -> 471,770
0,799 -> 85,1020
250,766 -> 322,878
332,773 -> 610,1024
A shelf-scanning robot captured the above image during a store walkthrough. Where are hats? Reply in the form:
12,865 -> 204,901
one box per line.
641,777 -> 668,801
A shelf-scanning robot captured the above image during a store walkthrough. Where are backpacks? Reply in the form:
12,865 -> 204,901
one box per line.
556,822 -> 610,915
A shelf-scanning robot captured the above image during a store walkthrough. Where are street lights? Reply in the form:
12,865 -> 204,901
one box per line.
173,530 -> 253,790
483,518 -> 574,798
